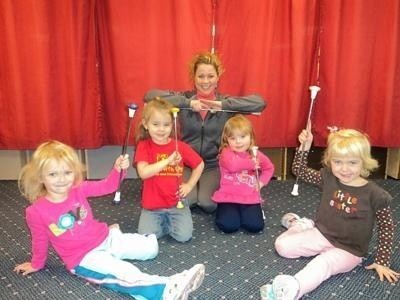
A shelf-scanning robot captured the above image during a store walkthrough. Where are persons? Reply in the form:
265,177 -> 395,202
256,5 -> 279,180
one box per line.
210,114 -> 275,233
13,139 -> 206,300
131,96 -> 205,243
260,128 -> 400,300
143,51 -> 268,215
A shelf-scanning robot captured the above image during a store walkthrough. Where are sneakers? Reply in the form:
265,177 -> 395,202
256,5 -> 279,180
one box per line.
278,211 -> 305,230
260,273 -> 300,300
163,263 -> 205,300
